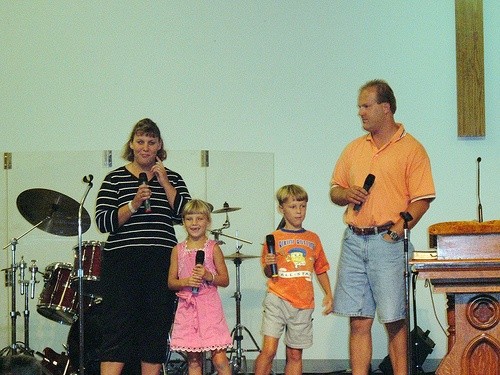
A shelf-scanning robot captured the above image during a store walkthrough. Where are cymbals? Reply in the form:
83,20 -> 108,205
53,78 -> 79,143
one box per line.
223,253 -> 262,260
16,187 -> 91,237
212,207 -> 241,214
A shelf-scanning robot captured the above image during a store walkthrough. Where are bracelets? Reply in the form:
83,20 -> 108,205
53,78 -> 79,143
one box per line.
206,273 -> 216,286
128,201 -> 137,214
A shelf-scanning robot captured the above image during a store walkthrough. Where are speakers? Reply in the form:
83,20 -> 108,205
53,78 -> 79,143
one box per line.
379,326 -> 436,375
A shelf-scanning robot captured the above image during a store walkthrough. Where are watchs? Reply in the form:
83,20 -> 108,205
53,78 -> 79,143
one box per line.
386,230 -> 400,241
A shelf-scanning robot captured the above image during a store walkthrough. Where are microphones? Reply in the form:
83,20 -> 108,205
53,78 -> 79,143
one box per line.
266,234 -> 278,278
193,250 -> 205,293
139,173 -> 152,214
476,157 -> 482,222
353,173 -> 374,212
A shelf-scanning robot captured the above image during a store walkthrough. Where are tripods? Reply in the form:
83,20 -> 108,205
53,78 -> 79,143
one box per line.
227,256 -> 261,365
0,213 -> 52,374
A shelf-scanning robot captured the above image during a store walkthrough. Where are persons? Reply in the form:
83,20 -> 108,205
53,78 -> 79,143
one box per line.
167,199 -> 231,375
333,79 -> 435,375
254,184 -> 333,375
95,117 -> 192,375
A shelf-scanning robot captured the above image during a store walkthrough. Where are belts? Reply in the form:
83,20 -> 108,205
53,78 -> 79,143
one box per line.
348,223 -> 393,236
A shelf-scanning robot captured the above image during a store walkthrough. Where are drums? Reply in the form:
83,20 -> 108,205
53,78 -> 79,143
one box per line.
65,303 -> 173,374
72,240 -> 107,296
36,261 -> 79,326
